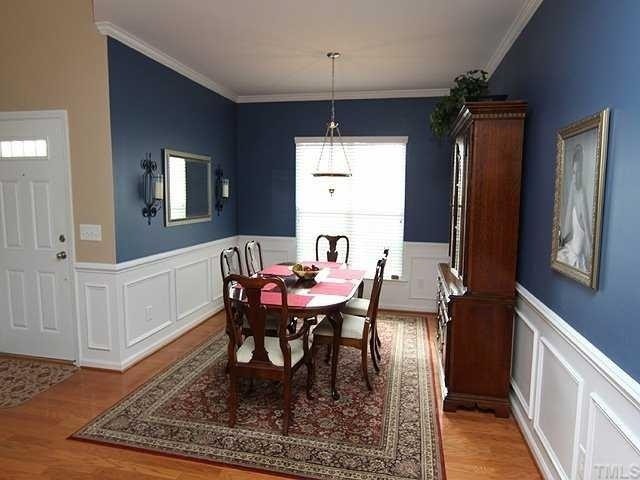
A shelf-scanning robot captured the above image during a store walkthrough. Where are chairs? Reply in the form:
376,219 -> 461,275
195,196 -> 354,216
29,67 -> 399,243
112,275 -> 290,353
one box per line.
218,234 -> 390,437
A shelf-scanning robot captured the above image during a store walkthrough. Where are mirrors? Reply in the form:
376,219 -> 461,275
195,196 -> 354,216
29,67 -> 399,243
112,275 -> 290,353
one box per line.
163,148 -> 213,228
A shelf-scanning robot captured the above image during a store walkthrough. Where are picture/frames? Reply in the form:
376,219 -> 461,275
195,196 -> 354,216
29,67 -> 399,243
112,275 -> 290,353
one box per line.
549,107 -> 610,292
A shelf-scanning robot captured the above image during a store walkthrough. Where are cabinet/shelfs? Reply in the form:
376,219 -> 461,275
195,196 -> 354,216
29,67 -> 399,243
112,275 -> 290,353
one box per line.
435,97 -> 532,419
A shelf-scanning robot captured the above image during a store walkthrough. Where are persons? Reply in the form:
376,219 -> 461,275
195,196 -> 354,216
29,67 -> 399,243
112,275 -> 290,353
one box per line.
558,142 -> 593,273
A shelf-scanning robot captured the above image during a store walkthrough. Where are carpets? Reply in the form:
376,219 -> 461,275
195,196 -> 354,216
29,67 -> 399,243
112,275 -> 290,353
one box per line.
66,310 -> 447,480
0,351 -> 79,410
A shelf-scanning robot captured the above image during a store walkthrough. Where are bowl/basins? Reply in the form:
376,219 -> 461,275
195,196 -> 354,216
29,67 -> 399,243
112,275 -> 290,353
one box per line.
291,262 -> 324,280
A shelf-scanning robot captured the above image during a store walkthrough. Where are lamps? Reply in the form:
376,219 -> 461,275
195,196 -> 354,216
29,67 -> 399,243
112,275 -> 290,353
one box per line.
215,163 -> 229,217
311,52 -> 354,198
141,152 -> 165,226
